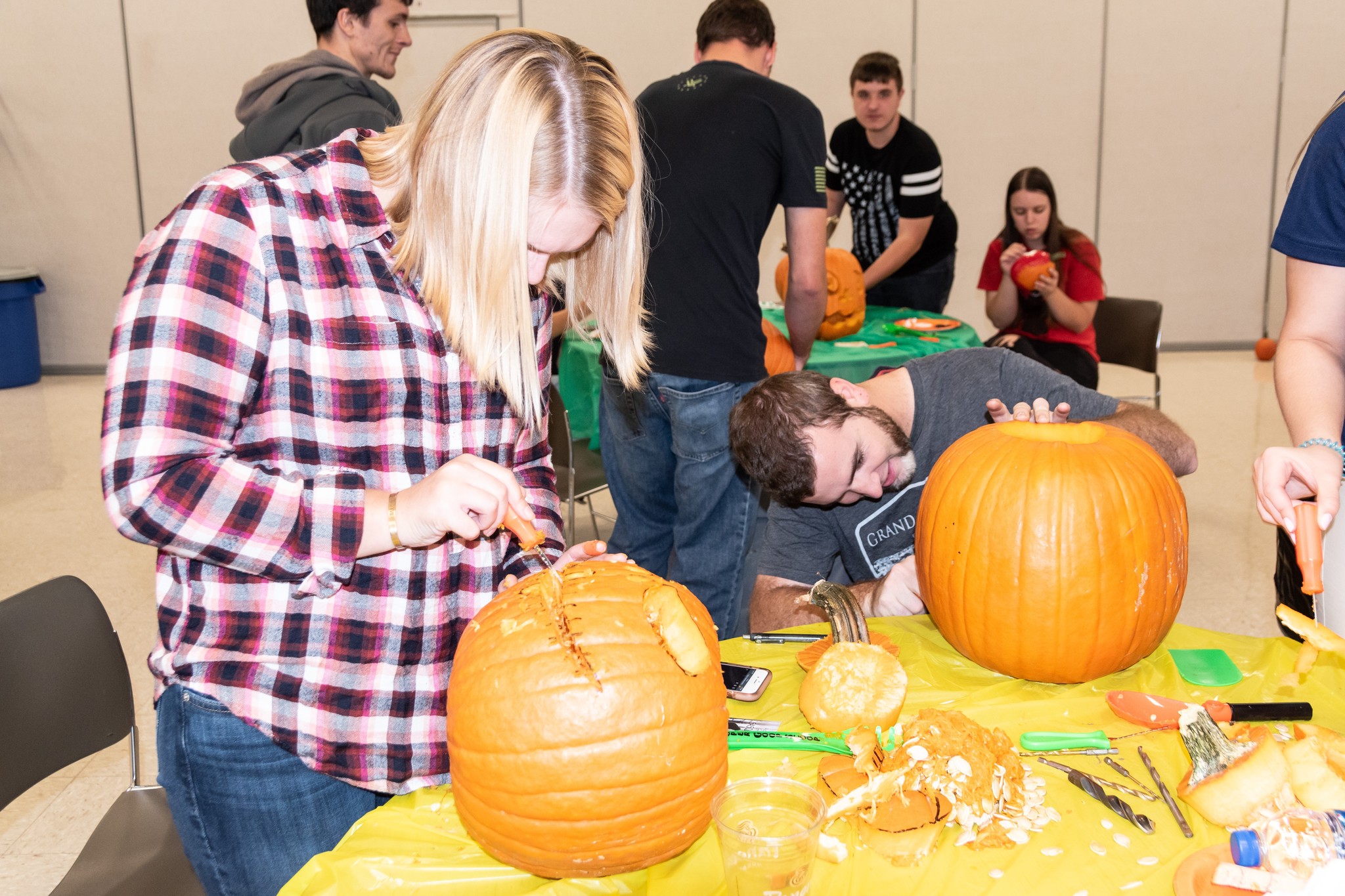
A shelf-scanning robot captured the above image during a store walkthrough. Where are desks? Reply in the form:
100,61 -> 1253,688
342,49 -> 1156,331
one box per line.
557,305 -> 985,452
271,612 -> 1345,896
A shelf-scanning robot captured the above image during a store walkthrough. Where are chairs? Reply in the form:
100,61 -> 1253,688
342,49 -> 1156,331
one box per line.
0,574 -> 218,895
539,384 -> 607,551
1091,296 -> 1164,414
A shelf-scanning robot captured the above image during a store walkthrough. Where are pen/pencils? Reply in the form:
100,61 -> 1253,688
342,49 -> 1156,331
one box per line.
742,632 -> 828,644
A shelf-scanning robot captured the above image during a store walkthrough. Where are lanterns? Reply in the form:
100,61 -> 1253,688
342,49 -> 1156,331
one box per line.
445,560 -> 729,881
776,241 -> 865,341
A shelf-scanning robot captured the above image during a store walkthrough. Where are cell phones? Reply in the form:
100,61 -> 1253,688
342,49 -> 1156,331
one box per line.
720,662 -> 773,704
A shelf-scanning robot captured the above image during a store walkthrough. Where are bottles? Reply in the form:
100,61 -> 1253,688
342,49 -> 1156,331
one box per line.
1230,810 -> 1345,872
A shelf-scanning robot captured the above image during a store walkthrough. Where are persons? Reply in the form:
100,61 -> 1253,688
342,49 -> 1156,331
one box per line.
552,279 -> 592,391
826,51 -> 958,312
228,0 -> 416,163
976,168 -> 1106,394
599,0 -> 829,634
96,28 -> 655,896
726,345 -> 1197,633
1252,92 -> 1343,639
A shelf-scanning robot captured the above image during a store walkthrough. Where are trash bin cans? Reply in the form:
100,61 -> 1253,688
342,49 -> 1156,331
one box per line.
0,266 -> 48,390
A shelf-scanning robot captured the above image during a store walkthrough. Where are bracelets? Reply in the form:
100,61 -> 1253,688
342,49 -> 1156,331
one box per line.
1296,438 -> 1345,480
388,491 -> 406,550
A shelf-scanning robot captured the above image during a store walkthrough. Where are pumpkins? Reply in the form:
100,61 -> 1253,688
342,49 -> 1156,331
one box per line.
912,420 -> 1190,684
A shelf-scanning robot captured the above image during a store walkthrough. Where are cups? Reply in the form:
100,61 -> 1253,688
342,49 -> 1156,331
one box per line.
710,774 -> 828,895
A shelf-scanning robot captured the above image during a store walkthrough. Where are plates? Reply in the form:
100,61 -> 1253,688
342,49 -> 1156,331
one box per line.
894,318 -> 961,331
